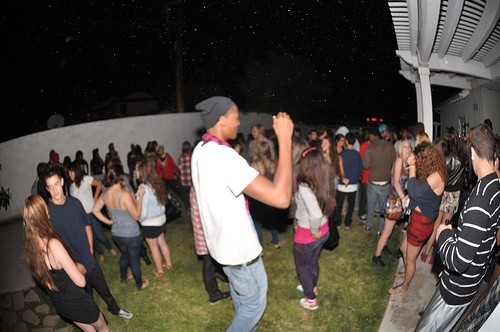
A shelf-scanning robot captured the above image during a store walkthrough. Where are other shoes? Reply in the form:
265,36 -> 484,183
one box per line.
336,225 -> 340,228
111,248 -> 117,256
395,248 -> 402,257
421,252 -> 428,263
383,245 -> 393,256
139,279 -> 149,290
372,254 -> 386,267
346,224 -> 352,230
162,264 -> 174,270
153,267 -> 164,277
127,275 -> 133,281
429,256 -> 435,264
273,242 -> 281,249
208,291 -> 231,305
360,213 -> 367,222
99,255 -> 105,263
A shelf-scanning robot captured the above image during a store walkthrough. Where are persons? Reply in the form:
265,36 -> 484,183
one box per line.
24,119 -> 500,332
190,97 -> 294,332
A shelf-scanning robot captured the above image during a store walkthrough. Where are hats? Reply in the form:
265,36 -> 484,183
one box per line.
195,96 -> 233,130
52,153 -> 59,162
378,124 -> 388,133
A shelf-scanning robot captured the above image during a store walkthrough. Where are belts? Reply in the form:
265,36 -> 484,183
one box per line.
234,256 -> 261,268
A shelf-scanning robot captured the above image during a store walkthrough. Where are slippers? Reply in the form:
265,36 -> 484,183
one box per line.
394,272 -> 405,278
388,285 -> 409,294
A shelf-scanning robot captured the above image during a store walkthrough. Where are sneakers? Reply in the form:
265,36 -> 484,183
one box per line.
299,297 -> 318,310
119,309 -> 133,319
296,285 -> 318,295
